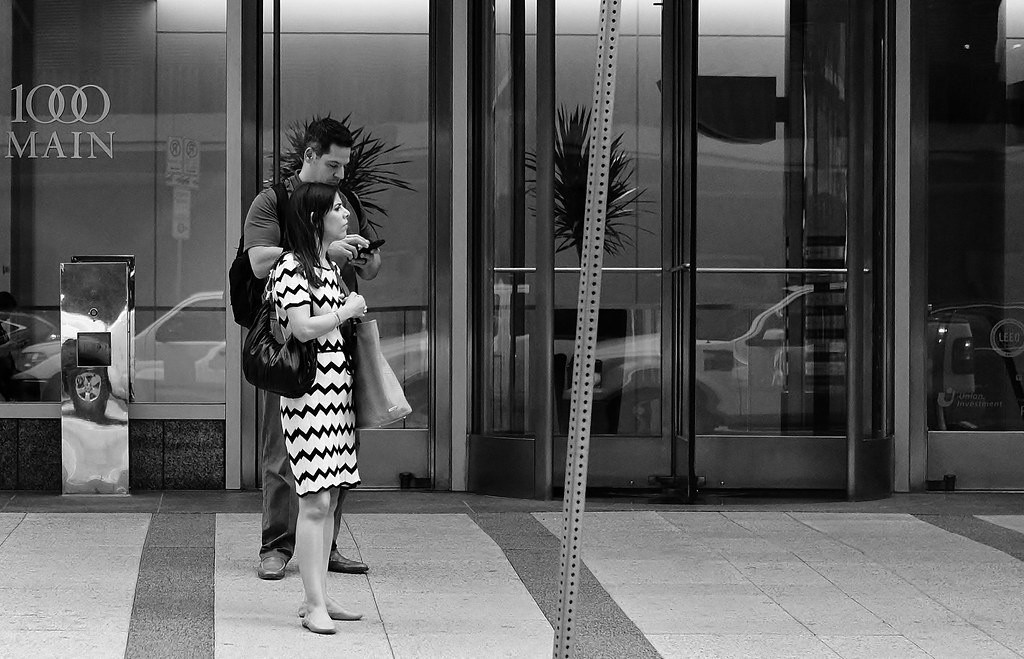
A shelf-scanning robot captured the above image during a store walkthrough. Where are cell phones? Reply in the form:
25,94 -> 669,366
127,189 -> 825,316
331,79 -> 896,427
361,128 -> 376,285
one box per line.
355,238 -> 386,260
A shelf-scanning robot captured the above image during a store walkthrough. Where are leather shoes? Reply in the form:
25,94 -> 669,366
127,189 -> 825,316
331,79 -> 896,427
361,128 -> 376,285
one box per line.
258,556 -> 287,580
330,551 -> 369,573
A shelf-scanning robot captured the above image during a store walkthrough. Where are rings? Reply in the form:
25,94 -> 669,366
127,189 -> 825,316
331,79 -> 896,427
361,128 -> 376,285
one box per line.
362,258 -> 367,265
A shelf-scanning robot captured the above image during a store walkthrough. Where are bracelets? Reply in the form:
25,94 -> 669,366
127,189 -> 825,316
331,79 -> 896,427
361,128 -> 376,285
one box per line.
331,311 -> 339,331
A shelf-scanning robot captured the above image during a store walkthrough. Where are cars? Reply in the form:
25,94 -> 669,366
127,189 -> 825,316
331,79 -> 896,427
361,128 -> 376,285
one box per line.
0,292 -> 575,429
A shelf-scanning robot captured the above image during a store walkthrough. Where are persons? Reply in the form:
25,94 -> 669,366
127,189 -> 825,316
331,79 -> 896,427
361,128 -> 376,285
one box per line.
262,181 -> 368,635
244,118 -> 382,580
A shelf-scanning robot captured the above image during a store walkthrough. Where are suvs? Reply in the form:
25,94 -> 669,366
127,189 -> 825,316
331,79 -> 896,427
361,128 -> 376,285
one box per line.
566,280 -> 856,435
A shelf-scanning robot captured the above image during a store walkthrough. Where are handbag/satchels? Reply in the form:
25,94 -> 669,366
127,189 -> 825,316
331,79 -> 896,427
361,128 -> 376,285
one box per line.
242,251 -> 316,398
330,263 -> 412,428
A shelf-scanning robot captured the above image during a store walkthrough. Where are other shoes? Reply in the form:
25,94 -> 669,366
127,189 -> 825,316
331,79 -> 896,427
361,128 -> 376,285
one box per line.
299,599 -> 363,619
302,616 -> 336,634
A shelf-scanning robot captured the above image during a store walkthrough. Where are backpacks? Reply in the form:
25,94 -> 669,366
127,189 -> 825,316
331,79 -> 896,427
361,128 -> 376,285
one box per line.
228,182 -> 362,331
